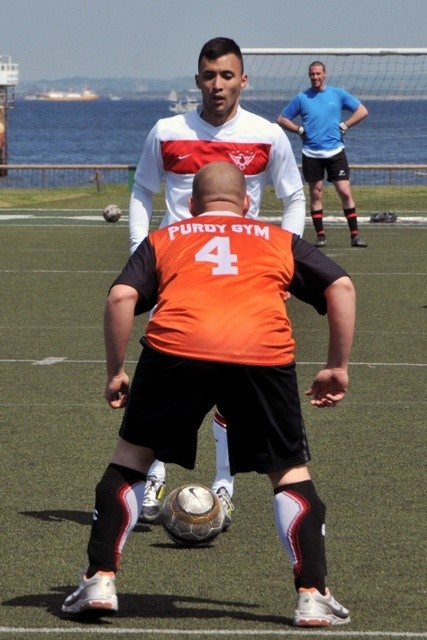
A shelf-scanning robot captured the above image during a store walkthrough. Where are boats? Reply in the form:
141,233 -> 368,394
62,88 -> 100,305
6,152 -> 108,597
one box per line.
169,96 -> 199,113
25,86 -> 98,101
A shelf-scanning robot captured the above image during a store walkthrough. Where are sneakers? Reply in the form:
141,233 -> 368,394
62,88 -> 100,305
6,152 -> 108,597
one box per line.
351,234 -> 368,247
61,571 -> 119,613
314,234 -> 327,247
138,474 -> 167,521
292,587 -> 351,628
216,487 -> 234,529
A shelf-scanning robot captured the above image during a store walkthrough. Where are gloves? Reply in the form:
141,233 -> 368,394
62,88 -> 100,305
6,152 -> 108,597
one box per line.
297,126 -> 304,139
340,122 -> 350,142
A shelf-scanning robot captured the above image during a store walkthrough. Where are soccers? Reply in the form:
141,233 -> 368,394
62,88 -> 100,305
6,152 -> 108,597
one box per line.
103,204 -> 121,222
159,483 -> 225,548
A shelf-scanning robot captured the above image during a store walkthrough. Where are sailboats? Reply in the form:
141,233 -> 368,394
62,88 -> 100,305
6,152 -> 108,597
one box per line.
167,89 -> 176,101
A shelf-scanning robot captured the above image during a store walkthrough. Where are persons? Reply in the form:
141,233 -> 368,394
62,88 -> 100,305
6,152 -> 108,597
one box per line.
128,38 -> 305,529
60,162 -> 356,629
277,61 -> 368,247
